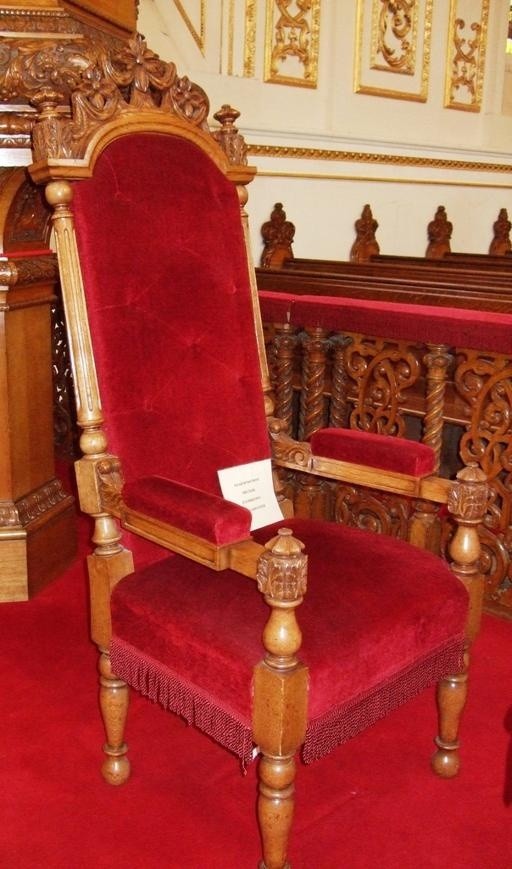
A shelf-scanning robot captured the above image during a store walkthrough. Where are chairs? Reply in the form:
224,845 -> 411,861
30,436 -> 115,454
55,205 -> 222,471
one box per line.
26,29 -> 490,869
